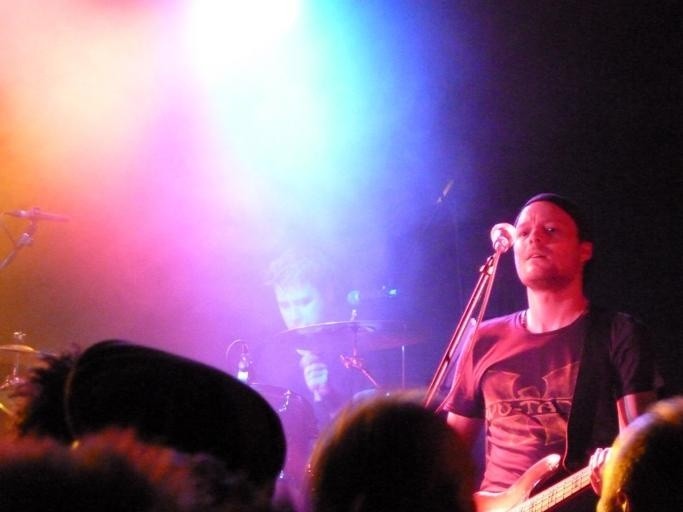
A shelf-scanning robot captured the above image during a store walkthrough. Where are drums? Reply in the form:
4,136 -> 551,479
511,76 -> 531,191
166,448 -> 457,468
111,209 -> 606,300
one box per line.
246,383 -> 317,494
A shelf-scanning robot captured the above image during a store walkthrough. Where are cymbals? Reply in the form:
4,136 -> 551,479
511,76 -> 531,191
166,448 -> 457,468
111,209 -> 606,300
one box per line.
272,318 -> 434,355
0,342 -> 69,368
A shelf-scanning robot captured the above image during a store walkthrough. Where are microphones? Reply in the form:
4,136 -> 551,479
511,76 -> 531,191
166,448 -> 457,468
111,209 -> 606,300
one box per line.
489,222 -> 517,252
15,208 -> 88,232
346,288 -> 398,305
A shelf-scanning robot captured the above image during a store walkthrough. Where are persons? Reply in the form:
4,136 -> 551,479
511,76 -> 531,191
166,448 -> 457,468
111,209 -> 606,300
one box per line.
596,396 -> 683,512
1,338 -> 287,511
251,259 -> 369,437
302,389 -> 478,512
443,191 -> 667,512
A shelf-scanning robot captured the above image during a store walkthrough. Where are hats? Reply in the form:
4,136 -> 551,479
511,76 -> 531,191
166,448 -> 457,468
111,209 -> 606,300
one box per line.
511,194 -> 593,243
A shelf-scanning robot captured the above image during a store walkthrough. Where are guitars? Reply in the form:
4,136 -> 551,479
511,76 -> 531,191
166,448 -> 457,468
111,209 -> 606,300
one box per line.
474,454 -> 596,512
